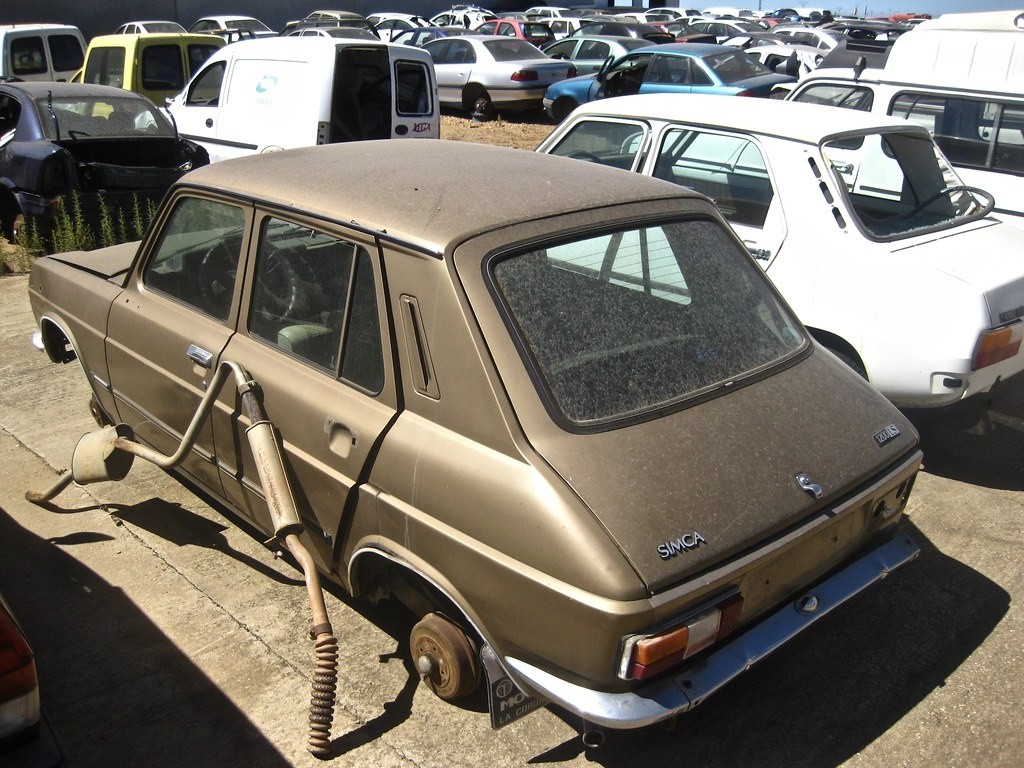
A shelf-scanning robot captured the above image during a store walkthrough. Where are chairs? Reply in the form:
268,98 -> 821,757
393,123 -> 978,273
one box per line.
277,275 -> 348,360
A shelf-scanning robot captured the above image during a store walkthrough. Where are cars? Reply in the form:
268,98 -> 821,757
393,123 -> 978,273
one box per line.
522,95 -> 1024,430
30,136 -> 931,749
1,0 -> 1024,248
